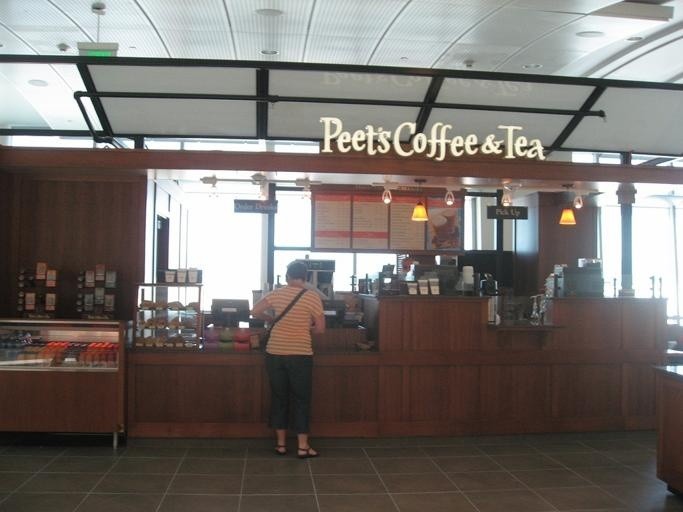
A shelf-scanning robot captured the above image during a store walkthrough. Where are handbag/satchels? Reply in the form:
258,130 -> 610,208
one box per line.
261,327 -> 271,345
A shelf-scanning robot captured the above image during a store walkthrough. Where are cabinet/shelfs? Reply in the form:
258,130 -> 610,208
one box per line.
15,266 -> 58,318
74,268 -> 122,321
131,282 -> 203,349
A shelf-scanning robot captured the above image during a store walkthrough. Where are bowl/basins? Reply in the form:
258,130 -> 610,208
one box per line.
356,341 -> 375,350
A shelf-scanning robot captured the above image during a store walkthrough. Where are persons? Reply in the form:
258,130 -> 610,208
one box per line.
252,262 -> 326,459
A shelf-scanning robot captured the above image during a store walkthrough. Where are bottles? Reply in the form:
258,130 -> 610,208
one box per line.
0,333 -> 119,368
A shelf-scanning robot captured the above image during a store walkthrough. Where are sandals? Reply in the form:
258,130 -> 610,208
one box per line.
297,446 -> 320,458
275,444 -> 287,455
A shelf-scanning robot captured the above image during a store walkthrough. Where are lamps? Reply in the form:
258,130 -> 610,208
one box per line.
200,172 -> 325,200
371,175 -> 601,226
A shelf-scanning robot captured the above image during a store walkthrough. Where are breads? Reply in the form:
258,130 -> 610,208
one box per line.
136,300 -> 198,347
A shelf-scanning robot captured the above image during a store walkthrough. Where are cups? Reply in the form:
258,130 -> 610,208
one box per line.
578,259 -> 586,268
